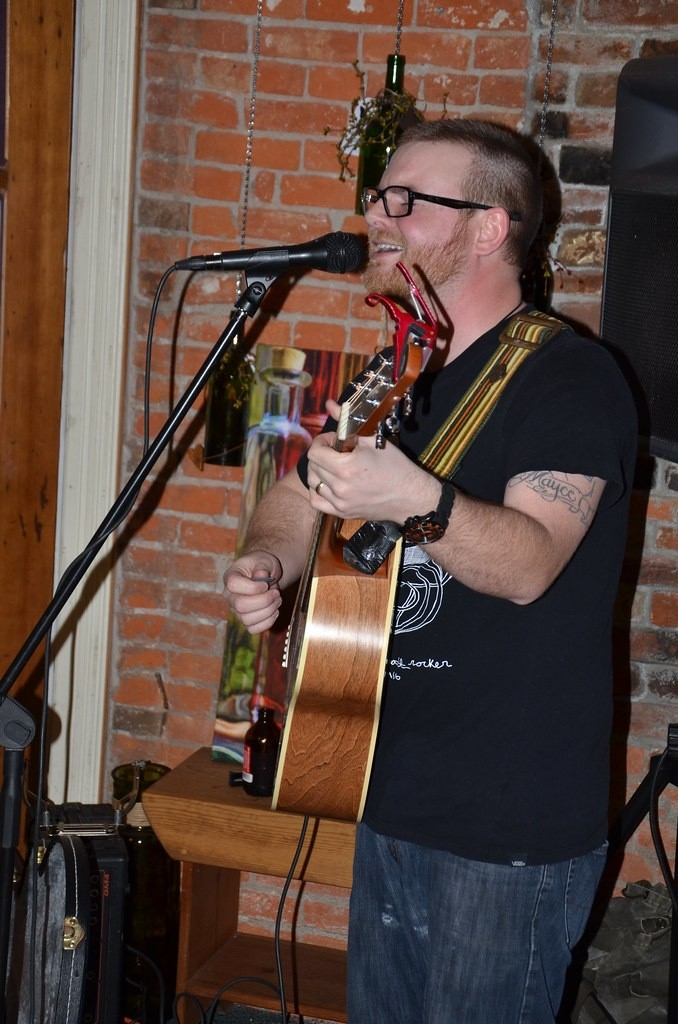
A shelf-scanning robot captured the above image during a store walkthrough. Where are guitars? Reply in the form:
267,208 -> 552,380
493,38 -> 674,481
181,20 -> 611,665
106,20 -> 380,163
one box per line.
269,261 -> 439,828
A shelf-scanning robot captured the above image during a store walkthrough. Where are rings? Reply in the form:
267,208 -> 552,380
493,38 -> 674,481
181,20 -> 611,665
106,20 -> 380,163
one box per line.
316,482 -> 322,494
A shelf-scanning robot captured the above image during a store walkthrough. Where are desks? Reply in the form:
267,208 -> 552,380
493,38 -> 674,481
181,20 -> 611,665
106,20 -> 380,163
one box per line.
143,747 -> 358,1024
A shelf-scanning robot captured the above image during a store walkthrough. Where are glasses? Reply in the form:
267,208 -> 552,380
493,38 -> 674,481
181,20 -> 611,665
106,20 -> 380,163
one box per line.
360,186 -> 522,221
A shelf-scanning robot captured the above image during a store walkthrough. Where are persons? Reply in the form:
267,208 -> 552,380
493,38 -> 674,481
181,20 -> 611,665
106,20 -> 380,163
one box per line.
225,118 -> 638,1024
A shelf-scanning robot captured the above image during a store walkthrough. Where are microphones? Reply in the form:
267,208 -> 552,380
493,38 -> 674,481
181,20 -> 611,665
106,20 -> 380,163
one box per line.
174,231 -> 362,274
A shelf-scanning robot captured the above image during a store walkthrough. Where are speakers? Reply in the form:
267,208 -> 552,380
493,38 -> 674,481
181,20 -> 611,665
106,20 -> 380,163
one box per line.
598,55 -> 677,463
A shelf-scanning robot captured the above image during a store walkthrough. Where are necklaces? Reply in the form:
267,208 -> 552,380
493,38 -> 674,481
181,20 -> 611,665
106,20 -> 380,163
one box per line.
502,301 -> 522,321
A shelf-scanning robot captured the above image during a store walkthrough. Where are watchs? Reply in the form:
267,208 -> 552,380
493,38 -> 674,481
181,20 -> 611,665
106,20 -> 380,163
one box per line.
404,482 -> 455,547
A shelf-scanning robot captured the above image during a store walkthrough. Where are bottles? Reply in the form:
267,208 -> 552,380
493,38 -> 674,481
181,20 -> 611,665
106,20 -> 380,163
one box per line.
242,707 -> 282,797
354,54 -> 414,216
